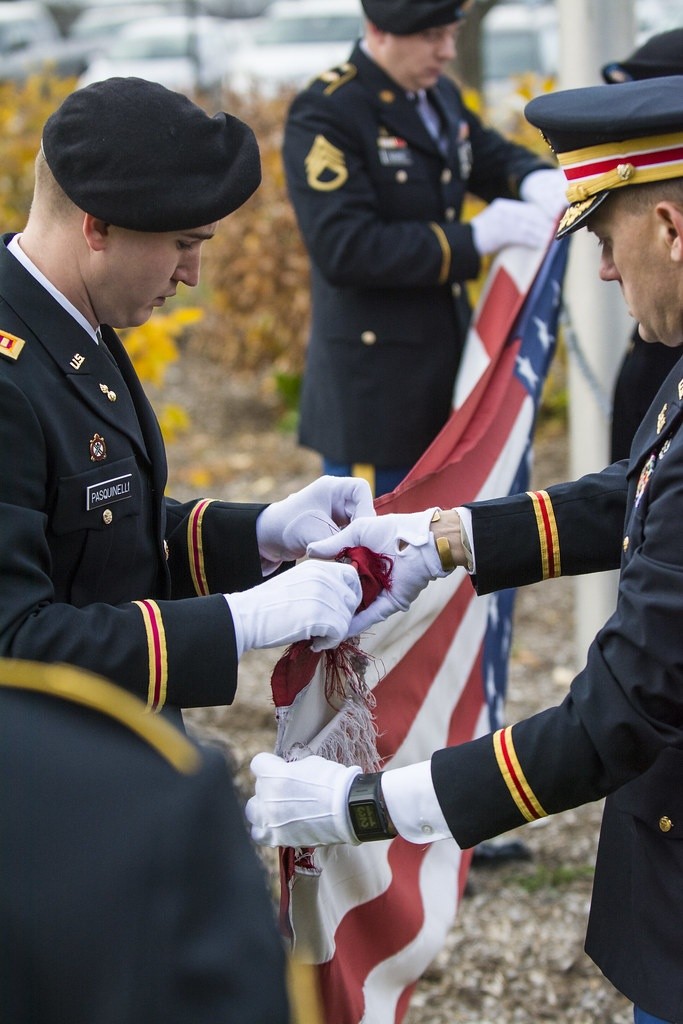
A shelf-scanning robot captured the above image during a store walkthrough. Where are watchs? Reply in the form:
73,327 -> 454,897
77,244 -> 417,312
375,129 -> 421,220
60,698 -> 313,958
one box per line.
346,771 -> 398,841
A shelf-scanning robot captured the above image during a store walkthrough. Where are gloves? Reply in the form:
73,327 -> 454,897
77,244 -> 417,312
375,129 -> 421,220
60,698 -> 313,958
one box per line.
470,198 -> 554,255
223,559 -> 363,659
519,169 -> 572,218
307,507 -> 457,641
256,474 -> 377,563
245,752 -> 362,848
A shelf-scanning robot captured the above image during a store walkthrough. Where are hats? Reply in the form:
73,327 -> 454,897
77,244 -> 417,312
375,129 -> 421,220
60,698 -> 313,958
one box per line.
524,75 -> 683,241
602,29 -> 683,84
361,0 -> 477,34
41,76 -> 261,233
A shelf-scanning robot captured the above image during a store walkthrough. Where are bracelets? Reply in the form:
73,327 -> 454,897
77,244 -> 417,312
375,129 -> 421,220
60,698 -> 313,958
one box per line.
430,513 -> 456,571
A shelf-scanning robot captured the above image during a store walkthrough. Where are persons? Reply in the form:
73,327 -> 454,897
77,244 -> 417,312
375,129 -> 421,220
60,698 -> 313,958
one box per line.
245,75 -> 683,1023
281,0 -> 683,895
0,80 -> 376,1024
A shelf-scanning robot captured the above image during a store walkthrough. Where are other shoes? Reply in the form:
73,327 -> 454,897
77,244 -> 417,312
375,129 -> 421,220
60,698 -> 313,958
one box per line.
473,840 -> 531,865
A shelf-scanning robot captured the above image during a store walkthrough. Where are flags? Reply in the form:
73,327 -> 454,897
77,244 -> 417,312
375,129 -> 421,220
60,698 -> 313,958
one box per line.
267,203 -> 575,1023
